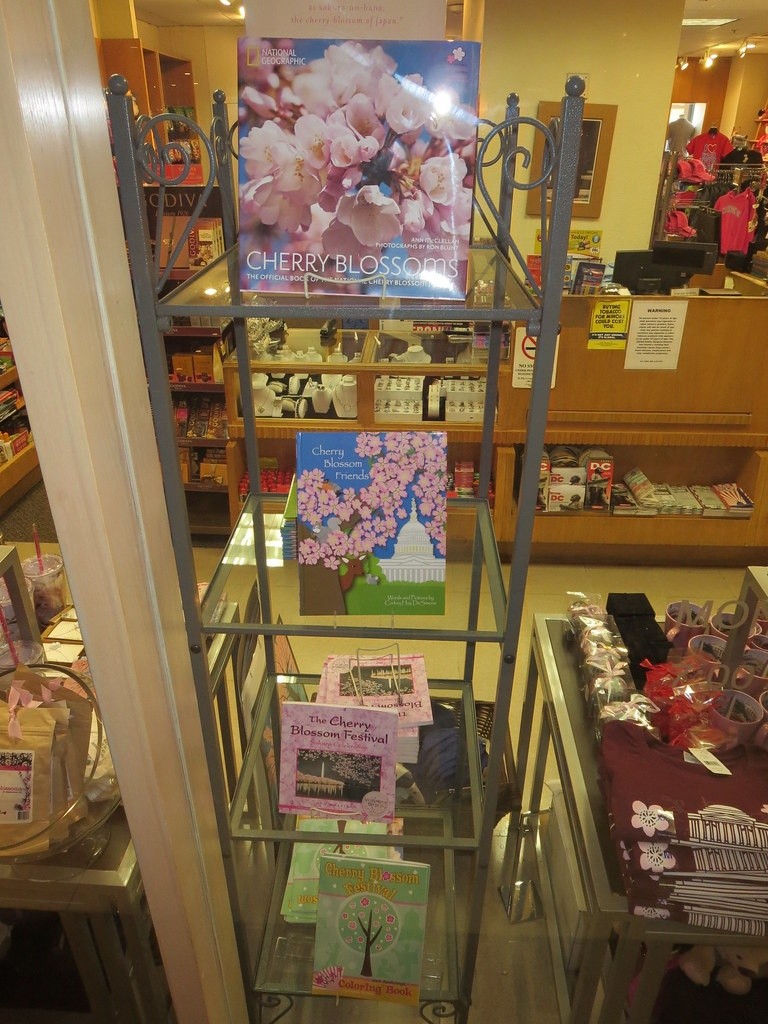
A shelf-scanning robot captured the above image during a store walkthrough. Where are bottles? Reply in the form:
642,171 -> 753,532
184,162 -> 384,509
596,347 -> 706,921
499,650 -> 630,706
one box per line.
0,638 -> 44,676
0,576 -> 35,644
18,553 -> 68,627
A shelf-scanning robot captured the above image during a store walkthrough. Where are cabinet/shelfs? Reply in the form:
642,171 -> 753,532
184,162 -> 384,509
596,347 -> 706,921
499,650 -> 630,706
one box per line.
0,366 -> 42,517
105,72 -> 587,1024
221,329 -> 509,564
128,268 -> 235,535
747,99 -> 768,150
100,38 -> 202,163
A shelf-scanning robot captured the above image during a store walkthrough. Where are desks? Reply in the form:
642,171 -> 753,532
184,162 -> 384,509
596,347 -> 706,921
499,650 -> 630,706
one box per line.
499,612 -> 768,1024
0,603 -> 266,1024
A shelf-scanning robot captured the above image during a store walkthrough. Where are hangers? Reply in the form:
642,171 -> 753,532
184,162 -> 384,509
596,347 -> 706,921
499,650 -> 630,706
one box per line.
709,166 -> 768,210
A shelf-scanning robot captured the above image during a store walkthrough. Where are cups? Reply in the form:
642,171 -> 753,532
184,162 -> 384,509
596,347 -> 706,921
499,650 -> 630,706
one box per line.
708,612 -> 768,703
707,688 -> 764,750
687,636 -> 728,680
664,600 -> 708,648
754,689 -> 768,751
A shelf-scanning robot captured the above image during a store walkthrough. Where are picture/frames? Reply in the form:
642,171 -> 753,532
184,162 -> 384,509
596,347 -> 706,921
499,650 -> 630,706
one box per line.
525,100 -> 619,218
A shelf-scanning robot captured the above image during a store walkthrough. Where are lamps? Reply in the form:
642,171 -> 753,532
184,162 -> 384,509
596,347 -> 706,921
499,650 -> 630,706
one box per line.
678,56 -> 688,71
699,48 -> 719,69
739,38 -> 756,59
